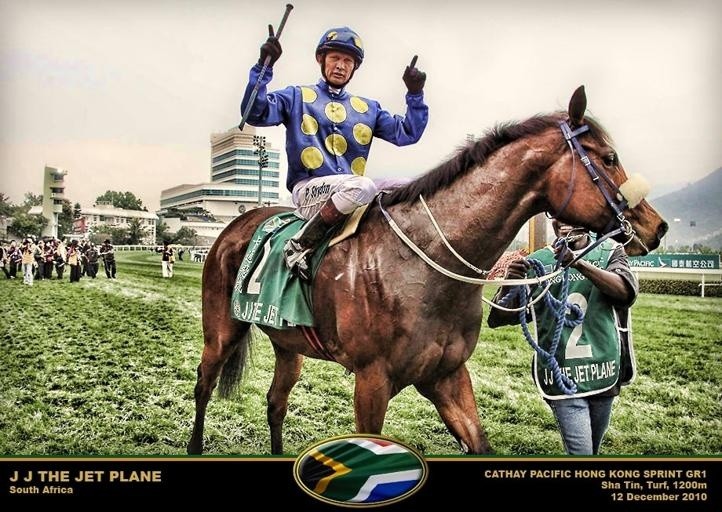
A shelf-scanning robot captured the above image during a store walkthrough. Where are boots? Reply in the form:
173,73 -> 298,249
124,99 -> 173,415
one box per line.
283,197 -> 350,284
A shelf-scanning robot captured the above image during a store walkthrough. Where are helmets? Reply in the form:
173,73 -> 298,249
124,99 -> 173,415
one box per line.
316,27 -> 364,64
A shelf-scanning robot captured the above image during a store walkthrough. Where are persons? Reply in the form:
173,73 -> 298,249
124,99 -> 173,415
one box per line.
178,249 -> 184,260
155,241 -> 172,278
169,247 -> 176,277
241,24 -> 428,281
487,217 -> 638,458
0,237 -> 116,287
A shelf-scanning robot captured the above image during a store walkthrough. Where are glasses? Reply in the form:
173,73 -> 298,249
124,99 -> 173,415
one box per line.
328,32 -> 363,49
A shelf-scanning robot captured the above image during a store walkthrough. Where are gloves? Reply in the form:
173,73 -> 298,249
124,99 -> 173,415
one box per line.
402,56 -> 426,93
258,26 -> 283,67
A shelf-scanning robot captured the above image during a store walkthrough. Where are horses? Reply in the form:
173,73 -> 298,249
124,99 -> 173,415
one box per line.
186,85 -> 669,456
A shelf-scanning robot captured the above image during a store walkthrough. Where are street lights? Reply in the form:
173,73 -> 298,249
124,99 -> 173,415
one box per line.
251,133 -> 270,208
